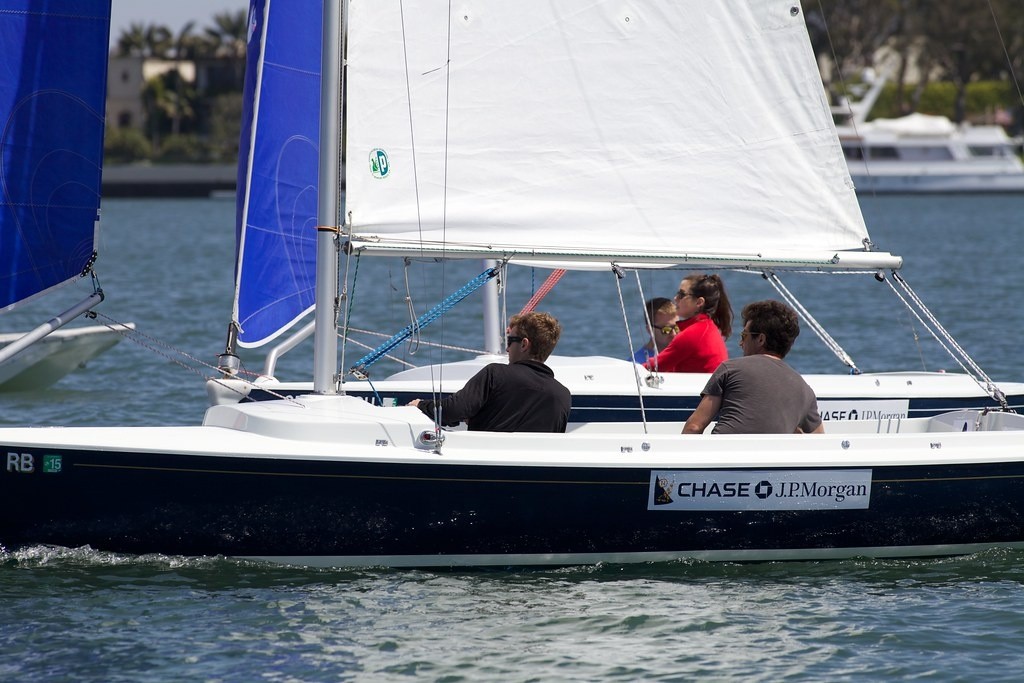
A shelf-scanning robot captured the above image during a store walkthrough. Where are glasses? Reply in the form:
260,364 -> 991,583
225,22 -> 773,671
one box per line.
507,336 -> 523,346
741,332 -> 767,344
678,292 -> 697,298
653,324 -> 679,334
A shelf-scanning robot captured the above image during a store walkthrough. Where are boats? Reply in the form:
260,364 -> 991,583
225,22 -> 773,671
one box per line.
835,111 -> 1024,195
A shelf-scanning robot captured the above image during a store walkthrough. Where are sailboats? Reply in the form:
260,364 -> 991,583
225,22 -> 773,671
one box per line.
0,0 -> 1024,566
0,1 -> 136,397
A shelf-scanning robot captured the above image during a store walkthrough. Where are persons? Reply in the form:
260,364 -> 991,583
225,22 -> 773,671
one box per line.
628,297 -> 679,364
682,300 -> 824,434
643,274 -> 734,374
407,312 -> 572,433
